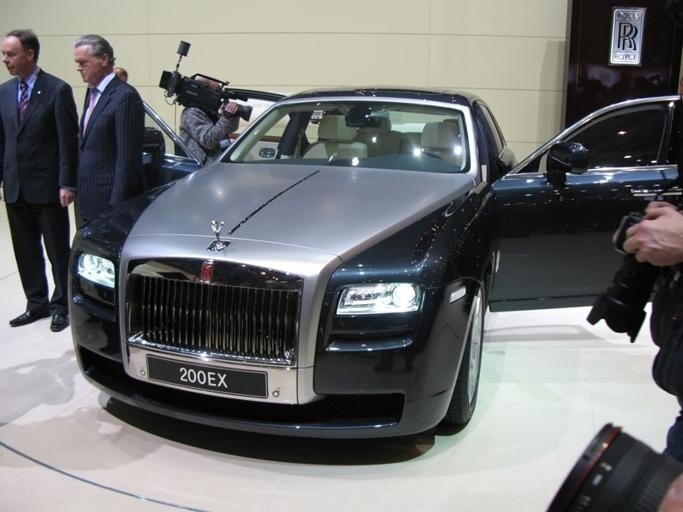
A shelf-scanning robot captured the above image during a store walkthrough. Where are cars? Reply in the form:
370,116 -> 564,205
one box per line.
63,76 -> 682,445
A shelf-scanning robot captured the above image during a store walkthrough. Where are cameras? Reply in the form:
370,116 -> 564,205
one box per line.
541,422 -> 683,512
587,212 -> 663,344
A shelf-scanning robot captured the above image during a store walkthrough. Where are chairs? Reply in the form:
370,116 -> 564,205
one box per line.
412,120 -> 462,169
298,113 -> 369,163
403,118 -> 461,157
354,114 -> 402,156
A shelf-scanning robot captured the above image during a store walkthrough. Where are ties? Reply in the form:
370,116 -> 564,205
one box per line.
18,81 -> 29,123
83,88 -> 98,132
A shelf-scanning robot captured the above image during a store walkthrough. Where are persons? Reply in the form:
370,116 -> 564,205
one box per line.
179,75 -> 240,169
114,67 -> 128,82
0,29 -> 79,332
622,201 -> 683,462
74,35 -> 149,232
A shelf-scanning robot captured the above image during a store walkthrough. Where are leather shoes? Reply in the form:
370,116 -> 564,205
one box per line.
50,307 -> 69,332
9,305 -> 51,326
